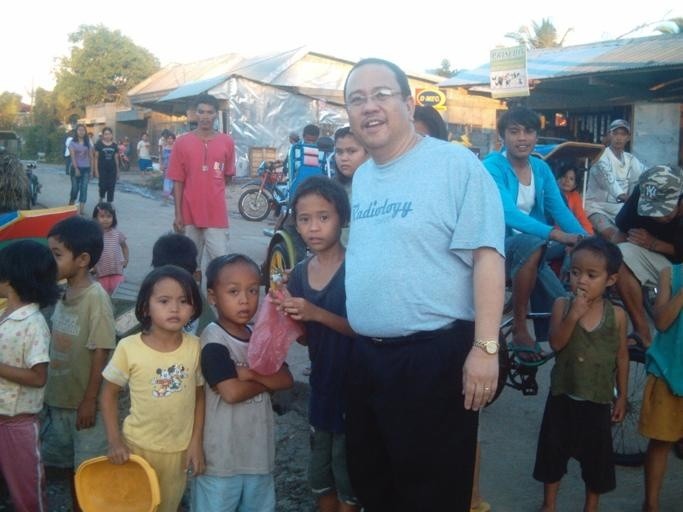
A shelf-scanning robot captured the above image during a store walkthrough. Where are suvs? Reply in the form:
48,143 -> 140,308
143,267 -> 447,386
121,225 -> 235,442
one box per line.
0,130 -> 42,210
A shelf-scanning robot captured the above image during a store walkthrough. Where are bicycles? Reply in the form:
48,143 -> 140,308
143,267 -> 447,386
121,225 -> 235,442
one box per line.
477,231 -> 656,468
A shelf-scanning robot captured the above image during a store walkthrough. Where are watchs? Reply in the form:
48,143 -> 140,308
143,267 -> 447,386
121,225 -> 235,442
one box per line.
472,338 -> 501,355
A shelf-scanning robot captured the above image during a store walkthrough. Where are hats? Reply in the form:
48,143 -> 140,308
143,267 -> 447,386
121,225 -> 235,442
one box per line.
636,164 -> 683,216
609,118 -> 630,133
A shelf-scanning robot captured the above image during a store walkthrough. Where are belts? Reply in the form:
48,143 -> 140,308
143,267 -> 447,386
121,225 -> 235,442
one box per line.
366,323 -> 472,347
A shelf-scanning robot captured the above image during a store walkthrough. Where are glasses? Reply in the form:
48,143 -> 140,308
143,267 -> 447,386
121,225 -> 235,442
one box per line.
346,89 -> 397,106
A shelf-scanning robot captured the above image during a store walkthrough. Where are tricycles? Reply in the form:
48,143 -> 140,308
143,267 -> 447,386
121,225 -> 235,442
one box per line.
257,142 -> 336,301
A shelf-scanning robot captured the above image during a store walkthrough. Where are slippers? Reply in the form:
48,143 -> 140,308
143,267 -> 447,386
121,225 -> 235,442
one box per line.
507,340 -> 545,367
626,334 -> 647,353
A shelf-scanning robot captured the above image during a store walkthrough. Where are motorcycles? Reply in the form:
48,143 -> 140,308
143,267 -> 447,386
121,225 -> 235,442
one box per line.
235,159 -> 288,222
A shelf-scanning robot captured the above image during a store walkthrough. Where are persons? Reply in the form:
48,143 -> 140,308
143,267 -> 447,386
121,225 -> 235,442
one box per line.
480,104 -> 683,512
344,58 -> 506,512
413,106 -> 447,142
64,123 -> 174,216
0,175 -> 351,512
168,95 -> 237,273
273,124 -> 319,175
